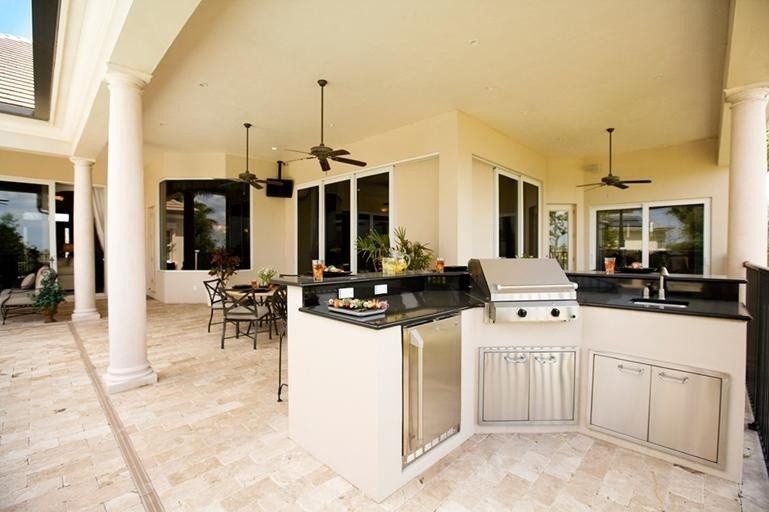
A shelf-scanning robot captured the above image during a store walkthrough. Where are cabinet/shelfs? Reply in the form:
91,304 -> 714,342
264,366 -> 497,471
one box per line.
286,276 -> 464,503
580,272 -> 753,484
464,259 -> 578,434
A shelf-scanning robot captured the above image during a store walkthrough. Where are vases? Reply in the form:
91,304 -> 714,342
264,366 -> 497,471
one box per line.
259,278 -> 270,286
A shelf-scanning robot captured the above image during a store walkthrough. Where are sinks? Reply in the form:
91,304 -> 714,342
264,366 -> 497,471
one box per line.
628,295 -> 689,311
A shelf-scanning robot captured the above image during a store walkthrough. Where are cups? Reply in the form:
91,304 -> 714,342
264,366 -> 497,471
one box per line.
435,256 -> 445,273
251,279 -> 260,290
382,257 -> 393,274
313,260 -> 324,282
604,257 -> 615,273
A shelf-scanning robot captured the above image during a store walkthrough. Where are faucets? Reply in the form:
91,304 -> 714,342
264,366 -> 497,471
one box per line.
656,264 -> 670,299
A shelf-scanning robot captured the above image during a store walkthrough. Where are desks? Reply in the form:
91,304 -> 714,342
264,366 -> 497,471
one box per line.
744,260 -> 769,477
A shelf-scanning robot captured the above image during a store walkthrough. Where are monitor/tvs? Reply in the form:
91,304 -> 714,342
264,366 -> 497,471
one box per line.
267,179 -> 293,198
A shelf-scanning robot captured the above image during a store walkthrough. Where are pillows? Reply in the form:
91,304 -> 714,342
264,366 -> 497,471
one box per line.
21,274 -> 35,290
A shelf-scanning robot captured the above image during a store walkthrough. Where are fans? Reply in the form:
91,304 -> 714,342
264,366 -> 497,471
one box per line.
214,124 -> 285,192
577,128 -> 651,193
283,79 -> 367,172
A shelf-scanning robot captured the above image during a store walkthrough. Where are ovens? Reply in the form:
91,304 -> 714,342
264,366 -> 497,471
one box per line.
402,311 -> 462,471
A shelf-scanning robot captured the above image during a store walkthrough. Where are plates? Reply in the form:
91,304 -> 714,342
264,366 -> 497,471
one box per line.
323,271 -> 352,277
619,267 -> 657,274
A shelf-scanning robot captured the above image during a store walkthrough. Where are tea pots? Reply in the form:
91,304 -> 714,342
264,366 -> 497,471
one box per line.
388,250 -> 411,276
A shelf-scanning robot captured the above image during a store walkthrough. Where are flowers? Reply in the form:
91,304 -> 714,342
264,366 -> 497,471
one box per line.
259,266 -> 277,281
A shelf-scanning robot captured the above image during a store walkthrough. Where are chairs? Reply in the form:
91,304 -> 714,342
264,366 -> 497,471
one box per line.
217,286 -> 273,349
203,278 -> 245,334
0,266 -> 57,325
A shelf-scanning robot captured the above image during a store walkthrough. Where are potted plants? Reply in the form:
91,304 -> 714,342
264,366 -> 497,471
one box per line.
206,245 -> 240,307
31,271 -> 68,323
